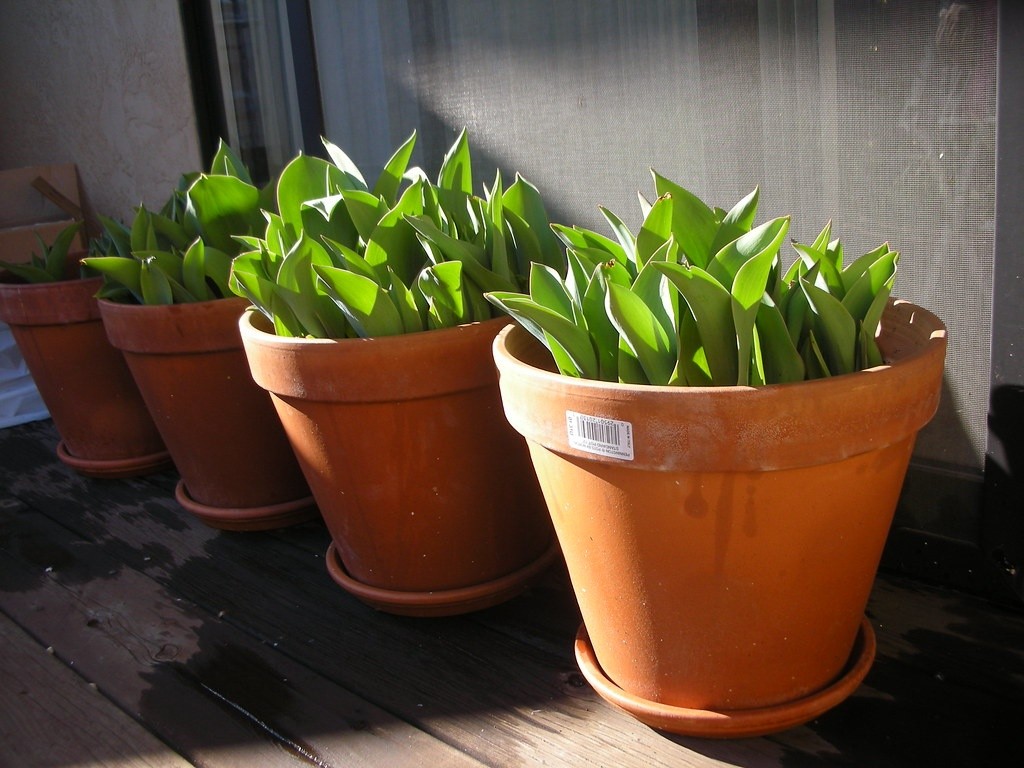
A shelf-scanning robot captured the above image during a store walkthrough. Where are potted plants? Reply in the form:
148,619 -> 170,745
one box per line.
226,124 -> 567,617
484,166 -> 947,737
79,136 -> 322,530
0,221 -> 176,481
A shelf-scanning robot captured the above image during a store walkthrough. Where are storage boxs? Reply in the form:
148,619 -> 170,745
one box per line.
0,163 -> 89,270
